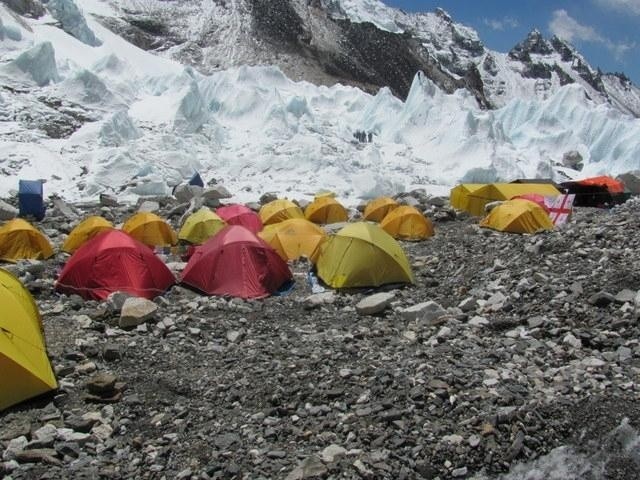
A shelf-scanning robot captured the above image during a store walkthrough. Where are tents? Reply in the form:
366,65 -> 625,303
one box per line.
0,265 -> 60,413
450,173 -> 639,236
0,217 -> 54,264
